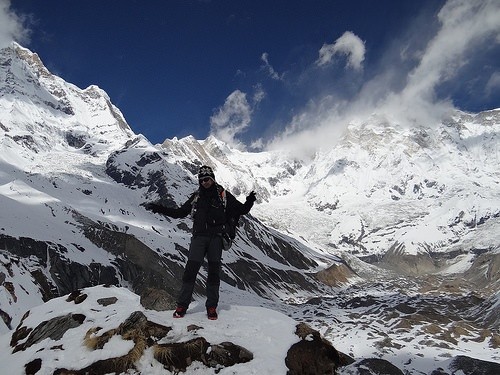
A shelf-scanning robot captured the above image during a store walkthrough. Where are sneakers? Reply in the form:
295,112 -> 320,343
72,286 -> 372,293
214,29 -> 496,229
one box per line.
173,307 -> 187,318
208,306 -> 217,319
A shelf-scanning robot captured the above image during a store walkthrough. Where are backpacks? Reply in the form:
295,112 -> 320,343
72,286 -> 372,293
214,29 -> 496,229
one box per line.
225,205 -> 241,240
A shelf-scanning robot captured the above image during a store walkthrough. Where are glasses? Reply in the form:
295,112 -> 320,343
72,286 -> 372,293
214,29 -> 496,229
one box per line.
198,177 -> 210,184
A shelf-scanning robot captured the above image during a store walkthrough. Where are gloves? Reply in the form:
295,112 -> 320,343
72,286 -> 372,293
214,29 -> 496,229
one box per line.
246,191 -> 257,203
143,203 -> 157,211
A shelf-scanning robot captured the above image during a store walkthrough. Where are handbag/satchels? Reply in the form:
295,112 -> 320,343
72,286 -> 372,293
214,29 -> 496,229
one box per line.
221,232 -> 232,250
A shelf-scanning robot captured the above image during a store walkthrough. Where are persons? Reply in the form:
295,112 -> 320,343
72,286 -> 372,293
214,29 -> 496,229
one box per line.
146,165 -> 256,320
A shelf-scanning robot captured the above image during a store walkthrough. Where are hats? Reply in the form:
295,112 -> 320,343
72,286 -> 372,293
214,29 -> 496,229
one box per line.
198,165 -> 215,182
